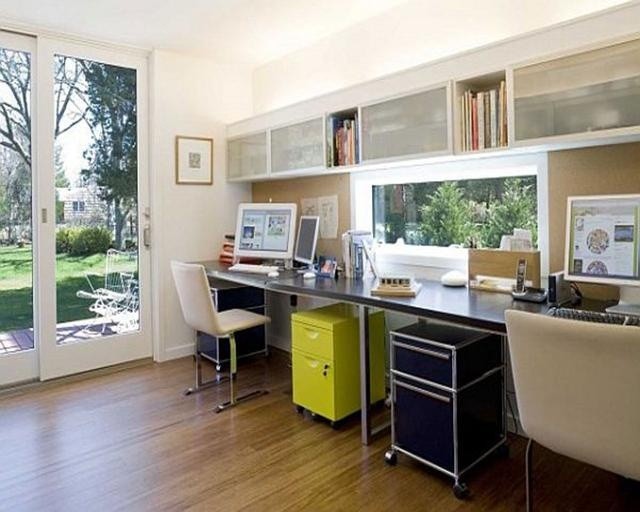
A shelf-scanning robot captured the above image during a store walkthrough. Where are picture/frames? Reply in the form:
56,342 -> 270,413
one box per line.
174,135 -> 215,187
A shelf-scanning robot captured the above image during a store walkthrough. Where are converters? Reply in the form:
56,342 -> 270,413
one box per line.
290,295 -> 297,306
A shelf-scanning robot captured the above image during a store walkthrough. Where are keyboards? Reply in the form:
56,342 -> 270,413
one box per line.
228,264 -> 279,274
545,306 -> 640,327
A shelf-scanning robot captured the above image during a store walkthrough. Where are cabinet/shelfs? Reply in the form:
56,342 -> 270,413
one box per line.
289,304 -> 388,427
385,322 -> 515,496
270,112 -> 327,178
453,31 -> 640,159
196,283 -> 268,366
328,81 -> 453,172
225,111 -> 270,180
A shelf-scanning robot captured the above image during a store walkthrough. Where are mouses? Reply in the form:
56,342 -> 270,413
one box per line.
268,271 -> 280,277
303,272 -> 316,278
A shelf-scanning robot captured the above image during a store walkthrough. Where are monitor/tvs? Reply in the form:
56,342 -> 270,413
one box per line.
233,202 -> 297,271
563,194 -> 640,315
295,215 -> 319,274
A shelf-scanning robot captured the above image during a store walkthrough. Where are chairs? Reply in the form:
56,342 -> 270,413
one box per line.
75,246 -> 138,331
166,262 -> 273,412
502,308 -> 639,509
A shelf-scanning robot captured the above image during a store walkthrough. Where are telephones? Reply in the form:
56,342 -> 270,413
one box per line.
512,259 -> 547,302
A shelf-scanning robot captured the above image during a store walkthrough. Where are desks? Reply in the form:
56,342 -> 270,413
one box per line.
197,259 -> 619,446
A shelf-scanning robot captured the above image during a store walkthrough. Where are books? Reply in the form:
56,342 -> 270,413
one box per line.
321,113 -> 363,169
217,231 -> 262,267
339,232 -> 364,281
451,80 -> 510,155
